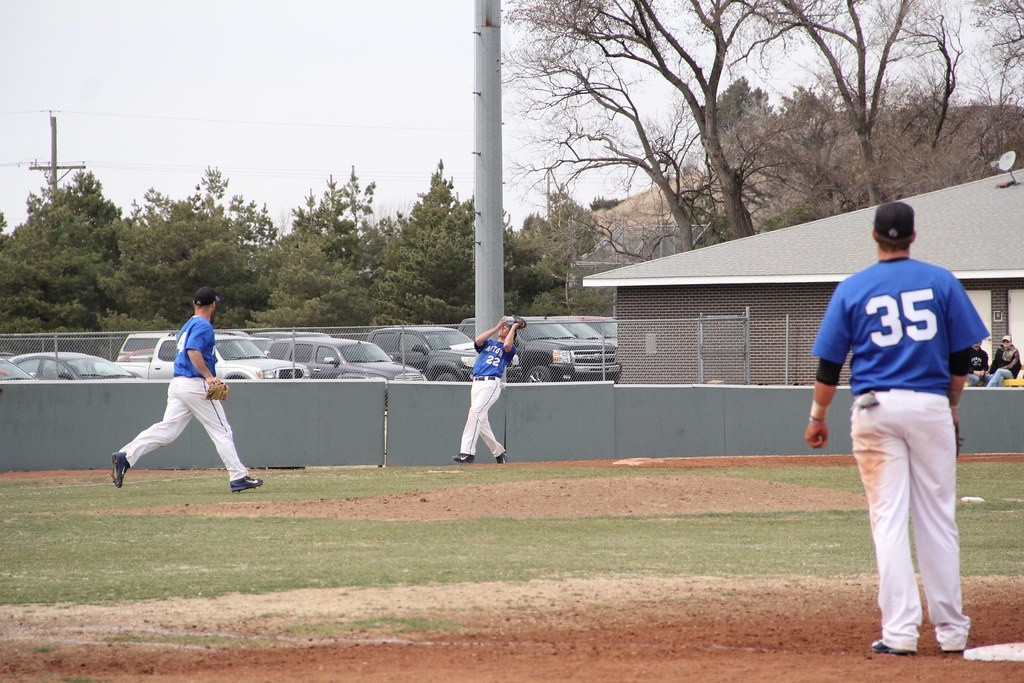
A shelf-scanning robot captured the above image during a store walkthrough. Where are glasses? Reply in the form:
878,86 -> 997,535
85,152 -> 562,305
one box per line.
1003,340 -> 1009,343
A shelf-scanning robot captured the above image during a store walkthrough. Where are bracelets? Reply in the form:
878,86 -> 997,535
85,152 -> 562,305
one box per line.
810,401 -> 828,418
951,404 -> 959,409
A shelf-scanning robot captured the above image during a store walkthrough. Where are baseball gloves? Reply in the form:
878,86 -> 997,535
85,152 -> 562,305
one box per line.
204,377 -> 230,402
513,314 -> 526,329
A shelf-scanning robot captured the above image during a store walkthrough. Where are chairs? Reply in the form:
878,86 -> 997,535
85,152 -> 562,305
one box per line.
1004,365 -> 1024,386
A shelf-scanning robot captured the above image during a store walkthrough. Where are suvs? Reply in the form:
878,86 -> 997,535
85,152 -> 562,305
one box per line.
366,325 -> 479,382
116,329 -> 310,380
458,315 -> 624,383
219,330 -> 429,382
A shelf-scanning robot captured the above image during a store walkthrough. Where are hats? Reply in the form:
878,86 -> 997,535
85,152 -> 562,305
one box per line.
874,202 -> 914,239
193,287 -> 221,305
1002,335 -> 1012,341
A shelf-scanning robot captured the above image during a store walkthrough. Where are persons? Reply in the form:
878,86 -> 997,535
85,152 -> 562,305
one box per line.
967,341 -> 992,386
805,203 -> 989,656
987,335 -> 1021,387
452,315 -> 527,463
112,287 -> 263,492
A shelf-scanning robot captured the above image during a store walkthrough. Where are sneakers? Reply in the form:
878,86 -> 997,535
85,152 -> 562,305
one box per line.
496,453 -> 508,464
230,476 -> 263,492
941,647 -> 961,655
110,451 -> 129,488
872,638 -> 914,654
452,453 -> 475,463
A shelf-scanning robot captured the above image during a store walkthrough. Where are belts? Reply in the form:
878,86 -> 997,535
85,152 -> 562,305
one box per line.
858,385 -> 891,396
475,375 -> 496,381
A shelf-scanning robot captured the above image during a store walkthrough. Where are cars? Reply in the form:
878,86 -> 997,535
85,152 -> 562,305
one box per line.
0,351 -> 145,381
